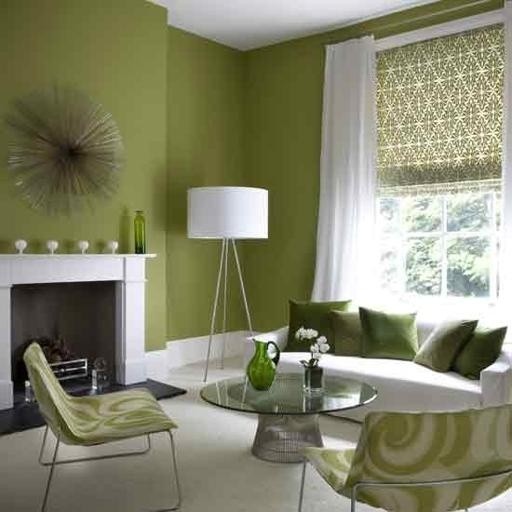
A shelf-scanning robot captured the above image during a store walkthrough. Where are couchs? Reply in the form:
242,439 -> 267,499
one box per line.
247,318 -> 512,427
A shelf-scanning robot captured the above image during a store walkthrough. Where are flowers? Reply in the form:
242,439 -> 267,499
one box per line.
294,327 -> 330,369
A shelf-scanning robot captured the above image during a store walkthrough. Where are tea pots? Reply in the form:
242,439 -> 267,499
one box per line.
246,339 -> 280,391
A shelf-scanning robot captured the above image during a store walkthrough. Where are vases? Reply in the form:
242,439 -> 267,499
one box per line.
304,365 -> 323,393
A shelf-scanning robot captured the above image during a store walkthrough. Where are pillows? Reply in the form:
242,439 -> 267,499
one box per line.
280,298 -> 509,381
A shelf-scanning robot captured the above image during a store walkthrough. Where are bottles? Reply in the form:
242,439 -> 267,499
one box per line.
134,209 -> 145,254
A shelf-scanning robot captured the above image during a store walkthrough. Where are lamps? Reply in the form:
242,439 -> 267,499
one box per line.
184,184 -> 269,388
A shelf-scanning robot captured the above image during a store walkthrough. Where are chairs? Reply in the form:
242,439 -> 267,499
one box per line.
298,403 -> 512,512
24,340 -> 182,511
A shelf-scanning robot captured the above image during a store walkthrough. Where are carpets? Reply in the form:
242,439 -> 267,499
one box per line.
1,377 -> 187,437
